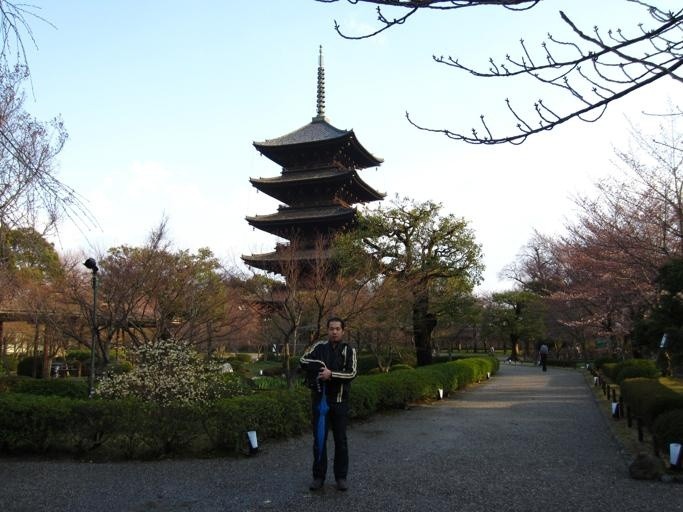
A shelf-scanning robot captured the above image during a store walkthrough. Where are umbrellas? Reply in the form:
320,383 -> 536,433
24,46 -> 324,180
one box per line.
312,375 -> 330,483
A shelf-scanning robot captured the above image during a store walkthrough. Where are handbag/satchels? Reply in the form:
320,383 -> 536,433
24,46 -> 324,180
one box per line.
303,367 -> 320,388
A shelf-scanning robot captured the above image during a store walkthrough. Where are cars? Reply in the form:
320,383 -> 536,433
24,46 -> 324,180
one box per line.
16,355 -> 77,379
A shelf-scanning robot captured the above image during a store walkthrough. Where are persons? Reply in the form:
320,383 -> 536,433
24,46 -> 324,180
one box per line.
538,342 -> 548,371
298,317 -> 357,492
488,345 -> 494,357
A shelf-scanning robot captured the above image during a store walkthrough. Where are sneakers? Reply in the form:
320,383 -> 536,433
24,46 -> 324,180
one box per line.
309,478 -> 324,490
336,479 -> 349,491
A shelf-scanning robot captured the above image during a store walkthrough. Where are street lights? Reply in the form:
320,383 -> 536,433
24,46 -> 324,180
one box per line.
79,256 -> 100,401
